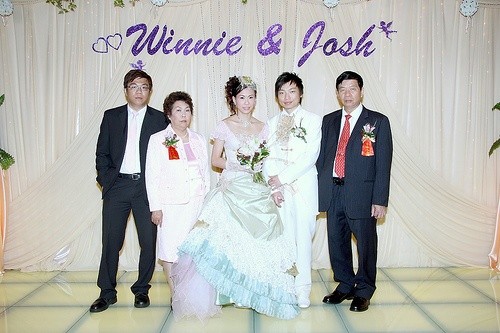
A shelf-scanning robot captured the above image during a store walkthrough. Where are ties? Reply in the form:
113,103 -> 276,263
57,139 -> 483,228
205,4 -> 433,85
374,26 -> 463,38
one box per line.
335,114 -> 352,179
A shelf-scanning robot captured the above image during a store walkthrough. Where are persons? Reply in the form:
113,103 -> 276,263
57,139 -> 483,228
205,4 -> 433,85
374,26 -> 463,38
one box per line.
90,69 -> 172,314
263,71 -> 322,309
169,75 -> 301,319
145,91 -> 210,310
314,71 -> 393,312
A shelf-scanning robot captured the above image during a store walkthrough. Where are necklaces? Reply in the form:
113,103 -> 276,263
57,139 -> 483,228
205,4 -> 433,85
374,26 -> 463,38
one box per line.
182,132 -> 187,139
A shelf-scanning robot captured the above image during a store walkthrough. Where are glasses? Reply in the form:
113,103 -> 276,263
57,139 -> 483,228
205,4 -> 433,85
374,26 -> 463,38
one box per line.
127,85 -> 149,91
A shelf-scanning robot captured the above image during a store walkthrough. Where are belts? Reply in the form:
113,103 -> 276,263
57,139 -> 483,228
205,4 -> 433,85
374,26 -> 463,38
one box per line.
333,178 -> 344,185
118,173 -> 141,180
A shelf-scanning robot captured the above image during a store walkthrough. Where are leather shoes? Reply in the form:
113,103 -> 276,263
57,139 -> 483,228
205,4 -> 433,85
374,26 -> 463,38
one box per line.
134,293 -> 149,308
90,294 -> 117,312
322,290 -> 354,304
350,296 -> 370,312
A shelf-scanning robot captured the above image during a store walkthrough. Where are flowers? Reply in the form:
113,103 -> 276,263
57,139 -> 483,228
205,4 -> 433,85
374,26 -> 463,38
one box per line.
162,129 -> 182,149
359,122 -> 377,143
235,134 -> 267,184
291,124 -> 306,142
